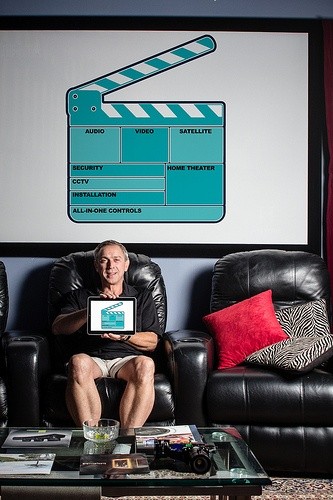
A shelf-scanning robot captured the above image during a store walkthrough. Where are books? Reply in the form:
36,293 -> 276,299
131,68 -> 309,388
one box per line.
0,425 -> 205,475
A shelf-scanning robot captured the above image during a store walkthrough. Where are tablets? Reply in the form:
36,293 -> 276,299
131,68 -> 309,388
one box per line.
87,296 -> 137,336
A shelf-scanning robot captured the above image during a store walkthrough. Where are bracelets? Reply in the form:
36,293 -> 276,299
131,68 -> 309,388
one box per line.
122,335 -> 132,343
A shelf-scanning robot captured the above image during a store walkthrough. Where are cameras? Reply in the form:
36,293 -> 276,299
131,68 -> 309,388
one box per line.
173,445 -> 212,475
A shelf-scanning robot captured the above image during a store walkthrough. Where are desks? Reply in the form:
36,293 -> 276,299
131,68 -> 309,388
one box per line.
0,424 -> 273,500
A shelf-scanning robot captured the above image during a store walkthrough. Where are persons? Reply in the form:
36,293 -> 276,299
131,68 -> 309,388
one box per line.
49,240 -> 164,436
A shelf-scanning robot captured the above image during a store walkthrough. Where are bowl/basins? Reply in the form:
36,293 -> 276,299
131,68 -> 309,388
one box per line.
83,418 -> 120,443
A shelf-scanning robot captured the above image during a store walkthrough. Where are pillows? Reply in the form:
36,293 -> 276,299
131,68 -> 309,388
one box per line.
201,289 -> 290,370
244,334 -> 333,374
275,298 -> 330,338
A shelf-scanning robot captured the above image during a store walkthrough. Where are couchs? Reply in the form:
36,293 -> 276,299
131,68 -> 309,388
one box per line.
0,250 -> 333,478
